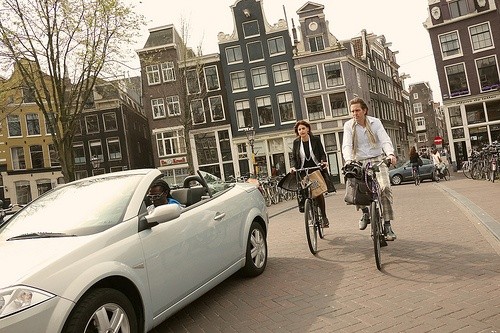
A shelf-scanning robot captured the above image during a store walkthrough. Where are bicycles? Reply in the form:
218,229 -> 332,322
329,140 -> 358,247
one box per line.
433,166 -> 450,183
293,162 -> 329,255
229,172 -> 298,207
462,142 -> 500,183
341,156 -> 396,270
412,166 -> 421,186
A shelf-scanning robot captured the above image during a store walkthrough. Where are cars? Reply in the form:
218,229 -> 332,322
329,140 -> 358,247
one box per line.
389,158 -> 436,185
0,168 -> 269,333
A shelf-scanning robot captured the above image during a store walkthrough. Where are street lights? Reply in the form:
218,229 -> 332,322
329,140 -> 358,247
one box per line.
244,124 -> 257,176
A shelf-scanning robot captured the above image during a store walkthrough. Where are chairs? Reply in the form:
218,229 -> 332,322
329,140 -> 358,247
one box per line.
169,175 -> 207,206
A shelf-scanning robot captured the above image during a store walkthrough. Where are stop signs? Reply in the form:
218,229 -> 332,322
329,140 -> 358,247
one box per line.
433,136 -> 443,144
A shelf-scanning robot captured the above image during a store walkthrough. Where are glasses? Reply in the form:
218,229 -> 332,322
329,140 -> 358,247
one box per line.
147,191 -> 165,200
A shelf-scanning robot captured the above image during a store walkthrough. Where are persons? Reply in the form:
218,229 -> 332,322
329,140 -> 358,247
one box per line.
289,121 -> 336,228
410,146 -> 423,181
342,97 -> 397,240
432,148 -> 446,175
146,180 -> 186,215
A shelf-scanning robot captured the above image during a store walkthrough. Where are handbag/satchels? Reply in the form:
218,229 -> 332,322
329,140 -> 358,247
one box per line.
303,170 -> 328,199
417,157 -> 423,166
344,177 -> 373,205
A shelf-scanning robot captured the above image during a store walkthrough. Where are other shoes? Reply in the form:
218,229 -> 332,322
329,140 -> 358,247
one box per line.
383,224 -> 397,240
359,213 -> 369,230
299,201 -> 305,213
321,215 -> 328,227
